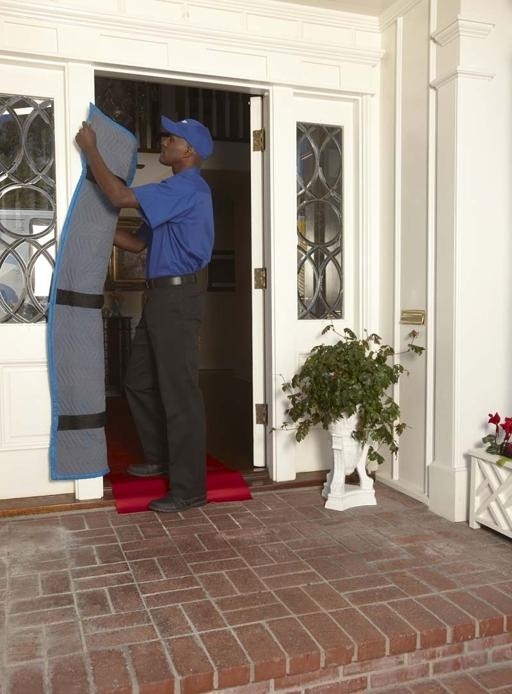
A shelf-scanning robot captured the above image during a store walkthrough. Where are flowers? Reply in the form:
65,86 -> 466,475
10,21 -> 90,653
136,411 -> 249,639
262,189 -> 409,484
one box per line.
481,412 -> 512,466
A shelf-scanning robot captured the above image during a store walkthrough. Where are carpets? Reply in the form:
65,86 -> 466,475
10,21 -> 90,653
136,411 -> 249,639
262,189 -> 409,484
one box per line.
105,395 -> 253,515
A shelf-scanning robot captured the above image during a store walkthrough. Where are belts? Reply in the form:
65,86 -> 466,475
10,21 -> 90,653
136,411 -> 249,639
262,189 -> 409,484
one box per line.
145,274 -> 195,289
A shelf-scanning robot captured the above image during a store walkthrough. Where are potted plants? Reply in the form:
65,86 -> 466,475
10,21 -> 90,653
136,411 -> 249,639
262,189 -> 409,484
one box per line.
267,323 -> 427,512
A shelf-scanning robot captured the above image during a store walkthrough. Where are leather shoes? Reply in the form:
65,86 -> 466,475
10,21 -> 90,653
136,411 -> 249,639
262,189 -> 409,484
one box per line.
127,461 -> 168,477
148,492 -> 208,513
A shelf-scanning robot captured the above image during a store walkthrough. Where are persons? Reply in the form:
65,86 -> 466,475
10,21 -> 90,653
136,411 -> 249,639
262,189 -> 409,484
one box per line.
75,116 -> 216,514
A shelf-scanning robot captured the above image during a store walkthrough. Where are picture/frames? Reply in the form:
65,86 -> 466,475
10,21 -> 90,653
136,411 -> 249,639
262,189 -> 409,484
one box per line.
103,216 -> 153,291
206,249 -> 235,292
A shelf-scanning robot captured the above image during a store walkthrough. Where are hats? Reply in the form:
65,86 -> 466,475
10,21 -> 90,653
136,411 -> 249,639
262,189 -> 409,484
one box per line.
160,114 -> 214,160
